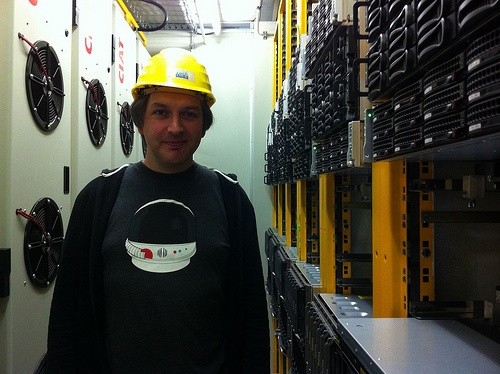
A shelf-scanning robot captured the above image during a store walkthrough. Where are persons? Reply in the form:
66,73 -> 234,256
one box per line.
34,48 -> 270,374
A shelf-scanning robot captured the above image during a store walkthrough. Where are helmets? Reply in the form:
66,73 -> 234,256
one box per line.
131,48 -> 216,108
